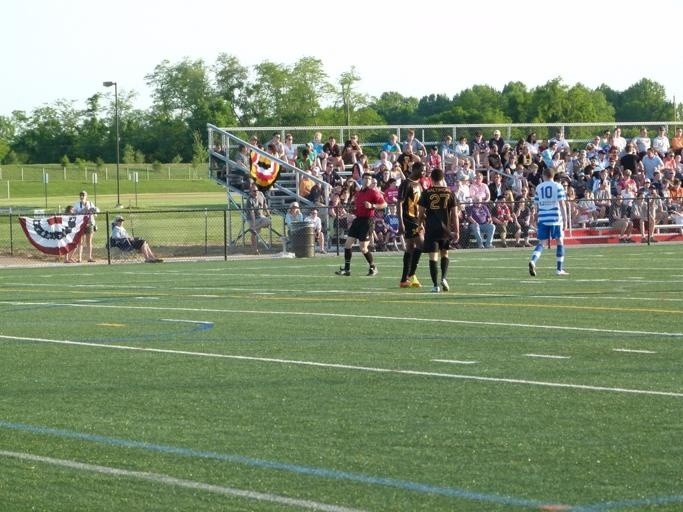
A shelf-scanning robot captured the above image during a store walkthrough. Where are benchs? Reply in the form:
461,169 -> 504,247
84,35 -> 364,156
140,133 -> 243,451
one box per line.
214,164 -> 374,210
559,218 -> 683,238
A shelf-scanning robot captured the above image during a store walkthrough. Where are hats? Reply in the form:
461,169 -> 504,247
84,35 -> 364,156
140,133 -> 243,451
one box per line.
116,216 -> 124,222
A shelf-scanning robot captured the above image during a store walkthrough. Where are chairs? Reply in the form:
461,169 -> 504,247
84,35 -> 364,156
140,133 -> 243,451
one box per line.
110,237 -> 143,263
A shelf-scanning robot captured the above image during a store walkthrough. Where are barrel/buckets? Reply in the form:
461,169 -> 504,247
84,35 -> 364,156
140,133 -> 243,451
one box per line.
290,221 -> 315,259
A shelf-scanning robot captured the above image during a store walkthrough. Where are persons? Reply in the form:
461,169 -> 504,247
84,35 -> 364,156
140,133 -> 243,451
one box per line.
395,160 -> 427,288
210,125 -> 683,255
110,214 -> 164,263
527,167 -> 570,277
417,168 -> 459,293
72,189 -> 99,263
60,204 -> 78,263
332,171 -> 388,276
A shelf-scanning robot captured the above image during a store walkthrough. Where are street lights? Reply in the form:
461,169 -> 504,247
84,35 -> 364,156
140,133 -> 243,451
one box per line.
104,82 -> 120,207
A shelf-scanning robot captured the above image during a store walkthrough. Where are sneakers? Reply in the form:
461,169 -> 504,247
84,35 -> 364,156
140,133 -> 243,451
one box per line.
529,261 -> 536,275
367,266 -> 377,276
431,287 -> 440,292
441,279 -> 449,291
335,270 -> 350,276
401,281 -> 419,288
406,275 -> 421,288
556,270 -> 569,275
